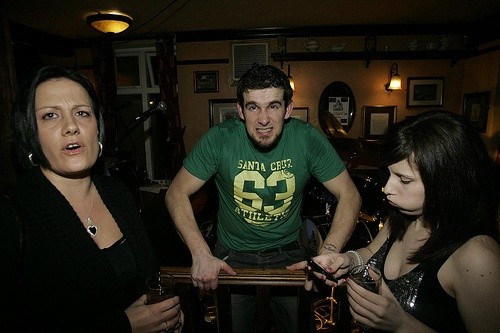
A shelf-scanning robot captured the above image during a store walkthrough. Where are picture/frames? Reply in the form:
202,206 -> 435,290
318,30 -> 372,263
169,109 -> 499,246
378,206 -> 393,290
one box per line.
291,107 -> 308,124
407,76 -> 445,109
363,105 -> 397,142
193,70 -> 220,93
461,91 -> 491,133
209,98 -> 239,128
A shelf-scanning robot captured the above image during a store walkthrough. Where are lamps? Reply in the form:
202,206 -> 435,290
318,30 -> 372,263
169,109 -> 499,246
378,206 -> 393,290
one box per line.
80,8 -> 134,35
386,63 -> 402,92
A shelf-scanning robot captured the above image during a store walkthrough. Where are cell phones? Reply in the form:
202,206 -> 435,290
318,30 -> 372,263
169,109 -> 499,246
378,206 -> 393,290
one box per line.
306,257 -> 338,284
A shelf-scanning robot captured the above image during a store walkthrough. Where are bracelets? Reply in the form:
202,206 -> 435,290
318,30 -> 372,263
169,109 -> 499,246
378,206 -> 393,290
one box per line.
346,250 -> 364,274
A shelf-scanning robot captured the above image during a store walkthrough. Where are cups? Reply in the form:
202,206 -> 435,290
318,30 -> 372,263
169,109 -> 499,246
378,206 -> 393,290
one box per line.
349,263 -> 383,331
407,38 -> 418,51
144,271 -> 176,305
384,45 -> 392,51
364,37 -> 377,52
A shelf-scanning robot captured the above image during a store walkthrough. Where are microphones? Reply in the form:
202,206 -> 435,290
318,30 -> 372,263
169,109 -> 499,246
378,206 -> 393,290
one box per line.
130,101 -> 167,122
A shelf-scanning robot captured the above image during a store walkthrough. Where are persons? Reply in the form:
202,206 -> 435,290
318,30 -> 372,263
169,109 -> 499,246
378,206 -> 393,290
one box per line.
164,63 -> 363,333
285,110 -> 500,333
1,64 -> 193,333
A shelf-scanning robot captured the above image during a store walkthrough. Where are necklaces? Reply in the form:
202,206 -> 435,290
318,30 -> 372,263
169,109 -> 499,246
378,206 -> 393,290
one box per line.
78,189 -> 98,236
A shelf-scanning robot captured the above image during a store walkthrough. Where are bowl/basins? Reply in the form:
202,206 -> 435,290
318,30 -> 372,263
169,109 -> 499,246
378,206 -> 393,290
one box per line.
424,44 -> 434,50
303,39 -> 322,52
331,46 -> 344,52
